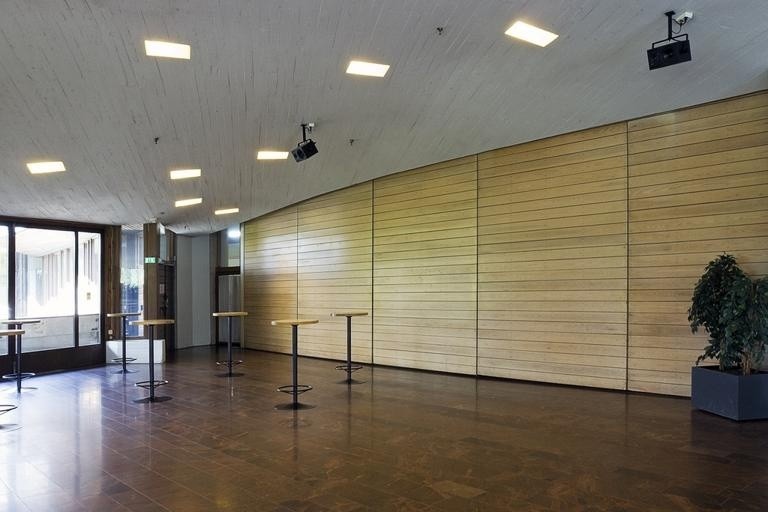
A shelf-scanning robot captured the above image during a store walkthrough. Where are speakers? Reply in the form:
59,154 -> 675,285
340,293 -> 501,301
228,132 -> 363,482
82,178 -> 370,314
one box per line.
292,142 -> 317,162
646,40 -> 692,71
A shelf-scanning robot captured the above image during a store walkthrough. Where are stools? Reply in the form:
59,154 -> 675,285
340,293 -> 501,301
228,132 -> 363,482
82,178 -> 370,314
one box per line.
329,312 -> 369,384
271,319 -> 319,411
213,312 -> 248,377
0,320 -> 41,414
107,312 -> 141,373
128,319 -> 176,404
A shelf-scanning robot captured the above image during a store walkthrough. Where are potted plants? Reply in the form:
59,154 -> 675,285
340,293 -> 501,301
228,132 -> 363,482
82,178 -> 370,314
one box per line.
686,252 -> 768,421
